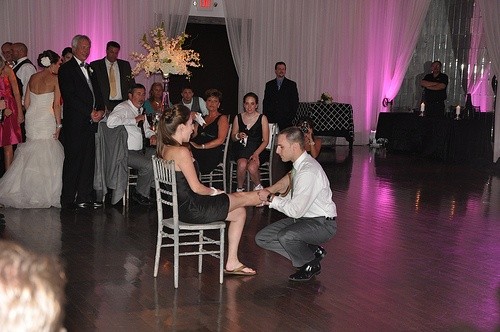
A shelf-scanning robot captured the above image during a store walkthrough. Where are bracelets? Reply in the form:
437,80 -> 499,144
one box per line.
202,144 -> 205,149
210,187 -> 218,197
55,124 -> 62,128
235,133 -> 240,141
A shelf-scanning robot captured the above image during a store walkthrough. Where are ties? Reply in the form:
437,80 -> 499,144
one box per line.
138,107 -> 148,149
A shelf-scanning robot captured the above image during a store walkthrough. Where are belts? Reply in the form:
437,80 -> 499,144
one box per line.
324,216 -> 336,220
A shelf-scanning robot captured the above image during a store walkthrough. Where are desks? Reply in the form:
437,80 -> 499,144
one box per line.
291,102 -> 355,152
376,111 -> 493,164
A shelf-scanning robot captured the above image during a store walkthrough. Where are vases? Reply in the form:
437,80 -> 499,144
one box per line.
161,77 -> 171,114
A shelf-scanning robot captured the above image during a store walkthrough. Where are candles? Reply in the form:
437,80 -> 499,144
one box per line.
421,102 -> 425,111
456,106 -> 460,114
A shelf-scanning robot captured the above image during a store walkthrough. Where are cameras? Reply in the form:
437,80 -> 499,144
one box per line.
300,122 -> 308,133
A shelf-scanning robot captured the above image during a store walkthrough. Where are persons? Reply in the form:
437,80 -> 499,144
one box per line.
255,127 -> 338,281
419,61 -> 448,112
158,103 -> 295,275
0,241 -> 74,332
297,117 -> 322,159
106,82 -> 229,207
262,61 -> 299,131
0,35 -> 135,208
230,93 -> 270,207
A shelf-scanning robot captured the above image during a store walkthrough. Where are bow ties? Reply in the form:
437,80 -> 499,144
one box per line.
80,62 -> 88,69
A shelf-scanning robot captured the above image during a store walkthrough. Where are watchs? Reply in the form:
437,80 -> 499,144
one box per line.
267,193 -> 276,203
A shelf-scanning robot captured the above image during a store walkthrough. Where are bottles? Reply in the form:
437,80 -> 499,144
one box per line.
465,94 -> 473,120
137,108 -> 146,127
0,96 -> 5,122
148,113 -> 153,130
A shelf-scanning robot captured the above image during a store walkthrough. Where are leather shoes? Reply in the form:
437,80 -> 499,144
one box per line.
288,260 -> 321,280
296,246 -> 327,270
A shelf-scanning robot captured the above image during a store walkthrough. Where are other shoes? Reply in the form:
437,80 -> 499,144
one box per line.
76,199 -> 103,208
133,192 -> 154,205
61,204 -> 81,211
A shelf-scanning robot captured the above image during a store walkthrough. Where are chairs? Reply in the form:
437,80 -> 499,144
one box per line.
96,122 -> 277,288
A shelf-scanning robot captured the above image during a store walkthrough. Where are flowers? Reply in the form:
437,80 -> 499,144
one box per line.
125,21 -> 203,83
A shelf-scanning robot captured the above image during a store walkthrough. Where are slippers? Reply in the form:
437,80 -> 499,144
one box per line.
281,172 -> 293,196
223,265 -> 256,275
